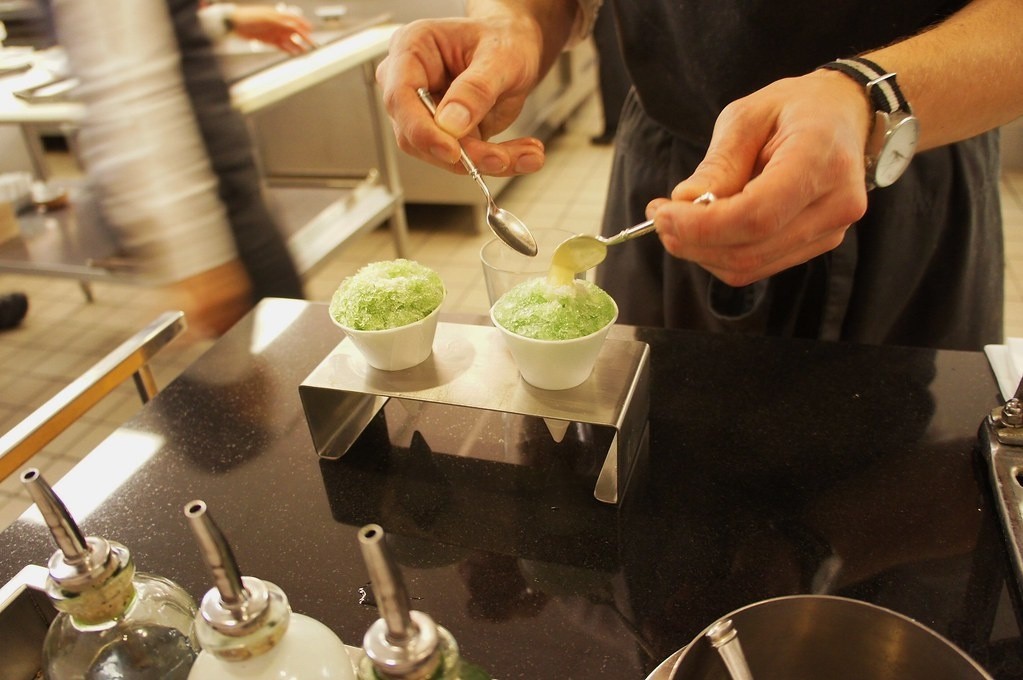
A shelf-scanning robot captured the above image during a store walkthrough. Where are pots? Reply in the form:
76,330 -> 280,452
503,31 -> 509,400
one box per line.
668,595 -> 990,680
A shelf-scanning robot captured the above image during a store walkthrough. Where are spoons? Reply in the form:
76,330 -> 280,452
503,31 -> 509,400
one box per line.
418,87 -> 538,257
552,193 -> 716,273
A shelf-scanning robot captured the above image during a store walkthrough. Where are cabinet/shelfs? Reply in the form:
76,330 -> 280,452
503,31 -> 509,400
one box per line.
0,28 -> 410,304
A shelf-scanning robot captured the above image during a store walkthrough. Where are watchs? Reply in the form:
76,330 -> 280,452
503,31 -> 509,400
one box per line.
822,55 -> 919,193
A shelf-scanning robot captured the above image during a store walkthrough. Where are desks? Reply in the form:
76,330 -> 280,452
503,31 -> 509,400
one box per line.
0,297 -> 1022,680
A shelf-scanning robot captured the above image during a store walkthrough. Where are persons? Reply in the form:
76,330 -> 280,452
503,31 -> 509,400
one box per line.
375,0 -> 1023,354
199,0 -> 318,56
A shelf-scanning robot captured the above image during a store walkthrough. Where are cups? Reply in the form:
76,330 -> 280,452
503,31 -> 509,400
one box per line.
479,228 -> 587,327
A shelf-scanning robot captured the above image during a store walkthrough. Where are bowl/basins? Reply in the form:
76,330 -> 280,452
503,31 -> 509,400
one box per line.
328,270 -> 446,371
489,279 -> 619,391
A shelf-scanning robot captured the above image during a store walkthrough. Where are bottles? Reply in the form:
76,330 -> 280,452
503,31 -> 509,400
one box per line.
22,469 -> 492,680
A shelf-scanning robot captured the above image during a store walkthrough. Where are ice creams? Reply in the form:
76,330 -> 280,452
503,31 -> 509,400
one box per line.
494,265 -> 617,342
328,254 -> 443,331
